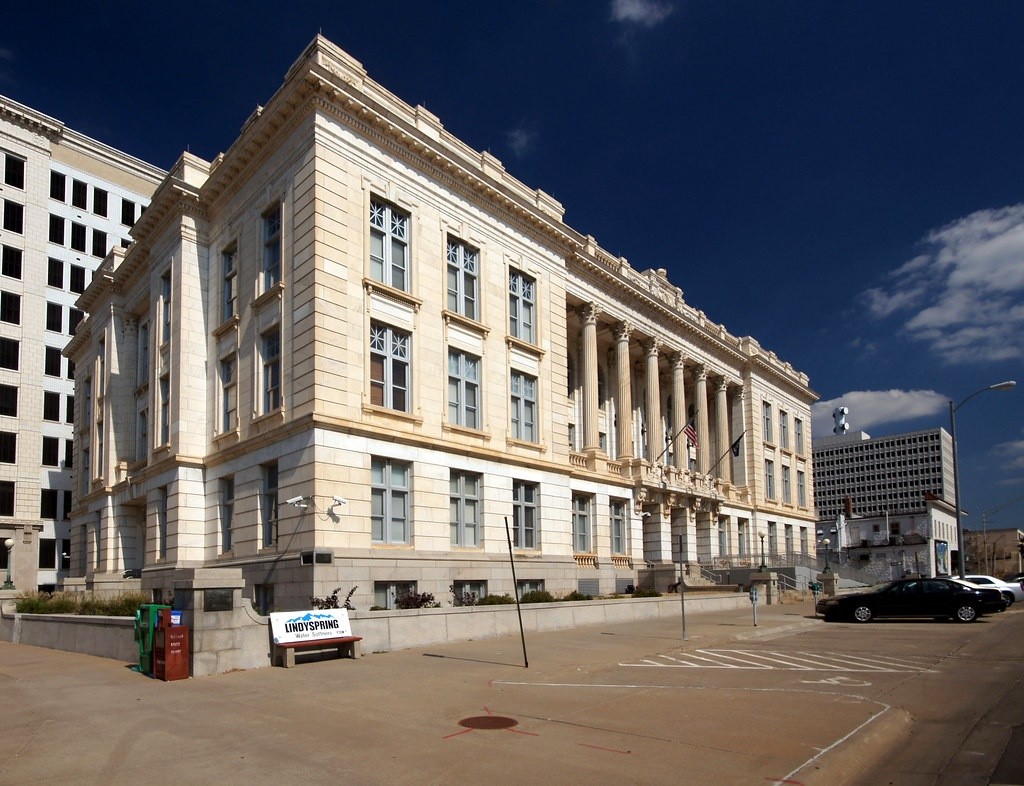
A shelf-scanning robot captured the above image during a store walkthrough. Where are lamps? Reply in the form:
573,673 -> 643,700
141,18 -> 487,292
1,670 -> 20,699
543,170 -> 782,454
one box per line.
758,529 -> 768,538
328,494 -> 347,514
822,537 -> 831,546
4,538 -> 15,550
286,496 -> 307,508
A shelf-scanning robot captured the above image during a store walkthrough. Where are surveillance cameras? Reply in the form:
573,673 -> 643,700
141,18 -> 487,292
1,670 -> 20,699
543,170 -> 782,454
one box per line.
333,495 -> 348,504
647,513 -> 651,518
62,553 -> 67,556
286,495 -> 303,505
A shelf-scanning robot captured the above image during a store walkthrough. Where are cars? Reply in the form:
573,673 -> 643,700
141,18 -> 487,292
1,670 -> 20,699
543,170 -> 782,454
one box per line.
816,575 -> 1024,624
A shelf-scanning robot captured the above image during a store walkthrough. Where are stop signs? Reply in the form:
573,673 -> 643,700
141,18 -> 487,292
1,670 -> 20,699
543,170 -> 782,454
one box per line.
750,585 -> 757,626
808,582 -> 819,619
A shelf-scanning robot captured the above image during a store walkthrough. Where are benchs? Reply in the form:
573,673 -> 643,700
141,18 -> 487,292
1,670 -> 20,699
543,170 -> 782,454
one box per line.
268,605 -> 363,671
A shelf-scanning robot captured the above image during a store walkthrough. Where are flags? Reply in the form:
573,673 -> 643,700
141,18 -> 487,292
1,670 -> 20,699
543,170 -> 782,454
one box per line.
732,435 -> 743,457
684,422 -> 700,450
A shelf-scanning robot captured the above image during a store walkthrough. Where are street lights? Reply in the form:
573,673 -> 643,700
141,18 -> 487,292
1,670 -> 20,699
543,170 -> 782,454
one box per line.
2,539 -> 16,590
759,531 -> 767,569
823,539 -> 831,570
950,381 -> 1016,580
